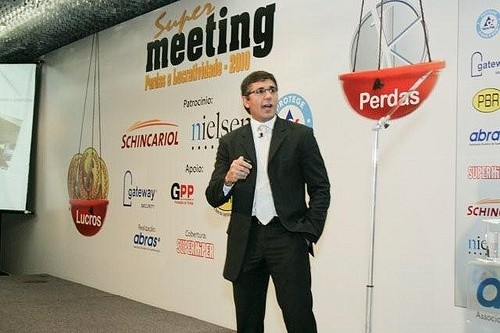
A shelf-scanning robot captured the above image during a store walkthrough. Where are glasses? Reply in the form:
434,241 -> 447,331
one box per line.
246,88 -> 277,96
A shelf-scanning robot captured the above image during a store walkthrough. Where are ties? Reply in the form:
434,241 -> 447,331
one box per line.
256,125 -> 275,226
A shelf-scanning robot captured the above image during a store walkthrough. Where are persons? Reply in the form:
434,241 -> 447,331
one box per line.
205,71 -> 331,333
